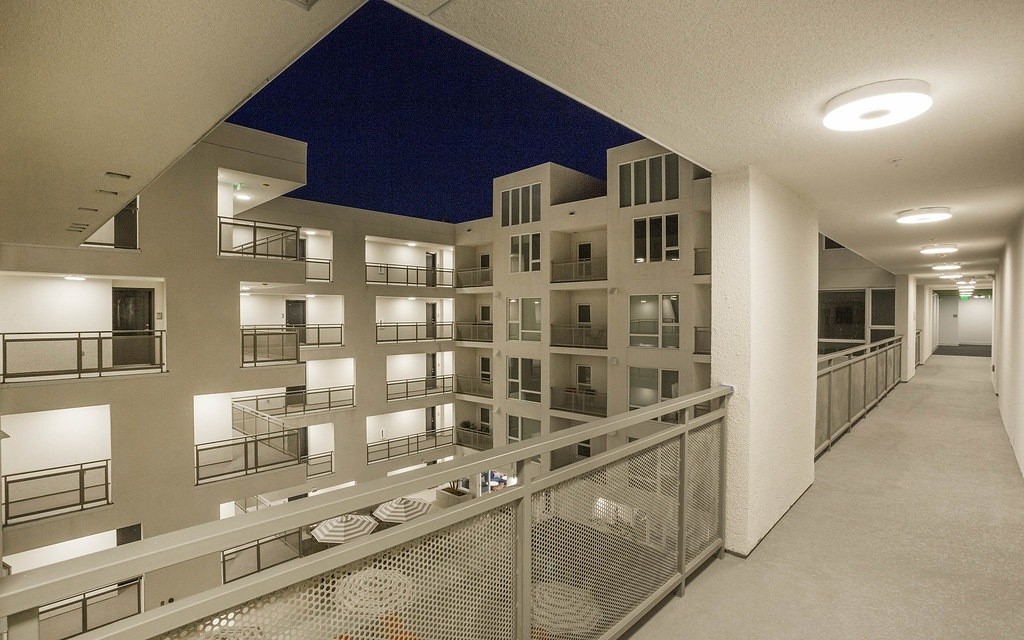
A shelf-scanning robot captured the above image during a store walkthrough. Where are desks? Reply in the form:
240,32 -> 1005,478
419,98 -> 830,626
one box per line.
486,481 -> 499,491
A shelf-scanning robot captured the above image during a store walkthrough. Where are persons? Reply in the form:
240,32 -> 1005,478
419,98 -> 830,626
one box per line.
510,474 -> 517,486
502,473 -> 507,485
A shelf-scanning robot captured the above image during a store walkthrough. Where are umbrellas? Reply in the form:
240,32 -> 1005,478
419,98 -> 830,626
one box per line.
374,497 -> 432,524
333,569 -> 416,620
530,581 -> 603,636
311,514 -> 379,543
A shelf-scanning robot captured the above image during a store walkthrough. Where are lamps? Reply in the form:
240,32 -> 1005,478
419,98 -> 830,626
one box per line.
938,274 -> 978,299
933,262 -> 963,270
920,244 -> 960,254
896,208 -> 953,225
65,274 -> 86,281
237,193 -> 252,200
823,79 -> 933,132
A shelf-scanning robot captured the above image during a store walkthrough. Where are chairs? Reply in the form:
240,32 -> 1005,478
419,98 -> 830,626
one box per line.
564,388 -> 577,405
592,328 -> 605,345
581,389 -> 595,406
